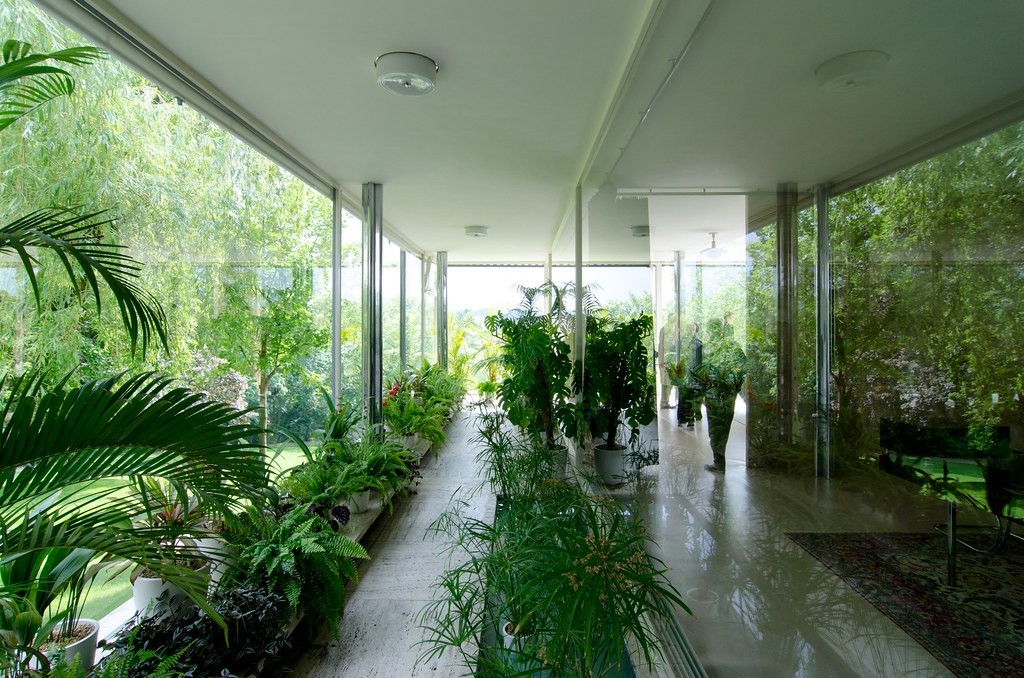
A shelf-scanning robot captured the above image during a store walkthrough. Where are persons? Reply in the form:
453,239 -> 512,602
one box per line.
657,312 -> 705,429
703,316 -> 749,474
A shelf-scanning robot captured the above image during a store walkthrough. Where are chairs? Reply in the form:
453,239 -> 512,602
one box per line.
934,448 -> 1024,553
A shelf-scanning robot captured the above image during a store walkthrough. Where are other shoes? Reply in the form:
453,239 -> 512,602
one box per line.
704,464 -> 725,475
687,422 -> 694,427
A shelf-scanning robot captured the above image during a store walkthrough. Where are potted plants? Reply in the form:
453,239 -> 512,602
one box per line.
408,279 -> 698,678
0,358 -> 472,678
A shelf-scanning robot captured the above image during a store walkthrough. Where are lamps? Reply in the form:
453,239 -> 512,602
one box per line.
816,50 -> 887,91
375,53 -> 437,96
632,227 -> 650,238
464,226 -> 486,238
